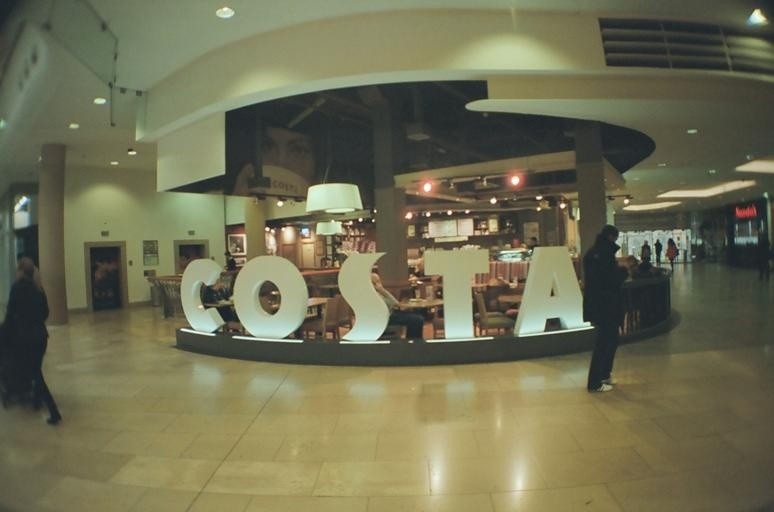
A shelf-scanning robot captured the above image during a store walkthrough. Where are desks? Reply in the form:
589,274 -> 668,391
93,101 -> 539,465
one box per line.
205,299 -> 232,307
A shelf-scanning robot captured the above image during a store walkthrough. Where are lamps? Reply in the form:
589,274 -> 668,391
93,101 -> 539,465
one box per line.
316,222 -> 342,236
306,169 -> 364,214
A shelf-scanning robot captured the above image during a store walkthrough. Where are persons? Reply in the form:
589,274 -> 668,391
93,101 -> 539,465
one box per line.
654,238 -> 663,264
582,223 -> 641,393
202,275 -> 245,334
526,237 -> 538,253
642,240 -> 650,260
0,256 -> 62,424
665,238 -> 680,266
223,251 -> 235,271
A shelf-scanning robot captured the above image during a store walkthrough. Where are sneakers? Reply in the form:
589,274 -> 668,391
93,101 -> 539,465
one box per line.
46,416 -> 62,425
588,383 -> 613,394
600,375 -> 618,386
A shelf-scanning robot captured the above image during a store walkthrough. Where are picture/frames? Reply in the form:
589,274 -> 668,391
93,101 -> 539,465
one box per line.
234,258 -> 246,267
228,234 -> 246,256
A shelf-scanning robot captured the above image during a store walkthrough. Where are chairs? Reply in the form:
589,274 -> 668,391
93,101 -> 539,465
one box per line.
226,276 -> 523,340
